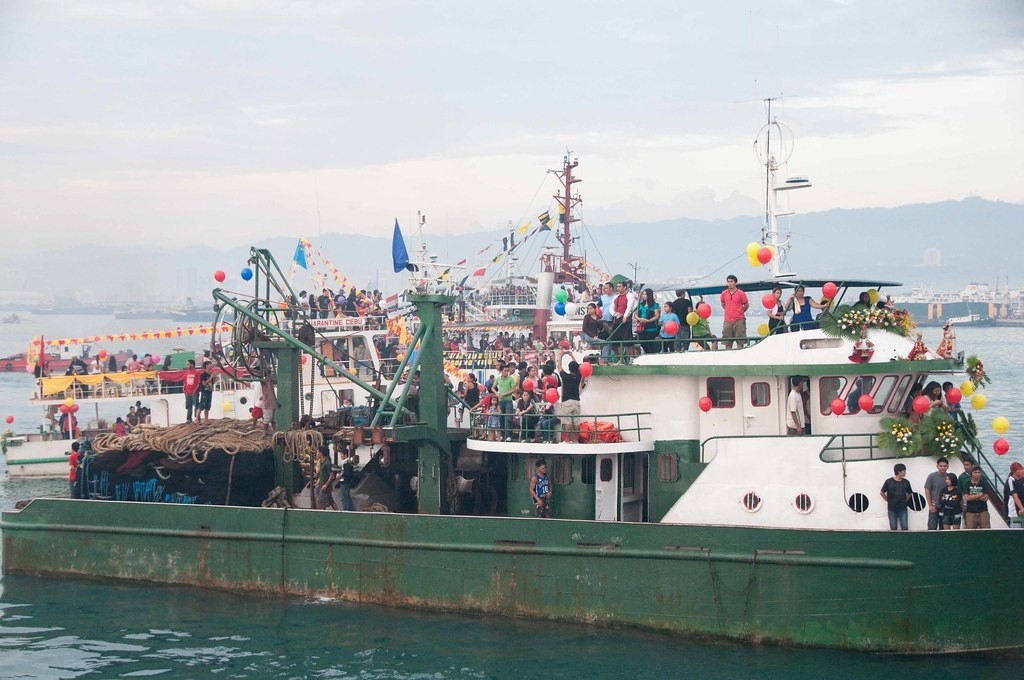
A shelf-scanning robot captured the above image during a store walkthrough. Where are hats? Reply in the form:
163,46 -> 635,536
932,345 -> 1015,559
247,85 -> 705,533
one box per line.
338,289 -> 345,293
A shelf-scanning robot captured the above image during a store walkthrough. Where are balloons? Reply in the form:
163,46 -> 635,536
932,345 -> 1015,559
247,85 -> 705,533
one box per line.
993,416 -> 1009,434
579,362 -> 592,377
912,396 -> 929,414
960,379 -> 986,413
686,243 -> 838,335
867,289 -> 879,305
858,394 -> 872,411
699,397 -> 712,414
831,398 -> 845,415
946,388 -> 962,406
521,380 -> 533,390
546,388 -> 559,403
994,439 -> 1010,456
5,265 -> 307,433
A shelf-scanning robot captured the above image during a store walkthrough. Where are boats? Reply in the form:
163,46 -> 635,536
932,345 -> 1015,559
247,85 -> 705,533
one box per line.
0,97 -> 1024,659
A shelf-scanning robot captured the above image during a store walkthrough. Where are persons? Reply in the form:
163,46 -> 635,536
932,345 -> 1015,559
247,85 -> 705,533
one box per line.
851,292 -> 870,309
821,378 -> 840,413
720,274 -> 749,349
903,382 -> 961,422
936,321 -> 956,359
847,376 -> 873,414
1004,462 -> 1024,522
787,375 -> 811,435
71,358 -> 582,518
877,301 -> 886,308
784,285 -> 830,333
880,457 -> 990,530
767,287 -> 789,335
34,278 -> 692,442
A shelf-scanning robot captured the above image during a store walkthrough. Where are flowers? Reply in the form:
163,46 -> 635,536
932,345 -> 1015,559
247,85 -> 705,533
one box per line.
822,304 -> 870,340
923,412 -> 965,458
967,356 -> 991,389
870,306 -> 915,334
880,416 -> 921,457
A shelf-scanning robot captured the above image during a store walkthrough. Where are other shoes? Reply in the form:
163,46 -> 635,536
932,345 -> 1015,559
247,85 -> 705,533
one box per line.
203,420 -> 211,425
194,421 -> 202,425
470,434 -> 581,444
186,419 -> 192,424
599,357 -> 634,366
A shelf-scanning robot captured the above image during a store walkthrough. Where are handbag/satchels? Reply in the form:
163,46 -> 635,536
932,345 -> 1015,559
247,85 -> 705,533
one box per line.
939,509 -> 945,518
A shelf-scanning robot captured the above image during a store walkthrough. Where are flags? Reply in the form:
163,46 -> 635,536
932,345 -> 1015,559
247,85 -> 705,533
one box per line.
392,223 -> 409,273
